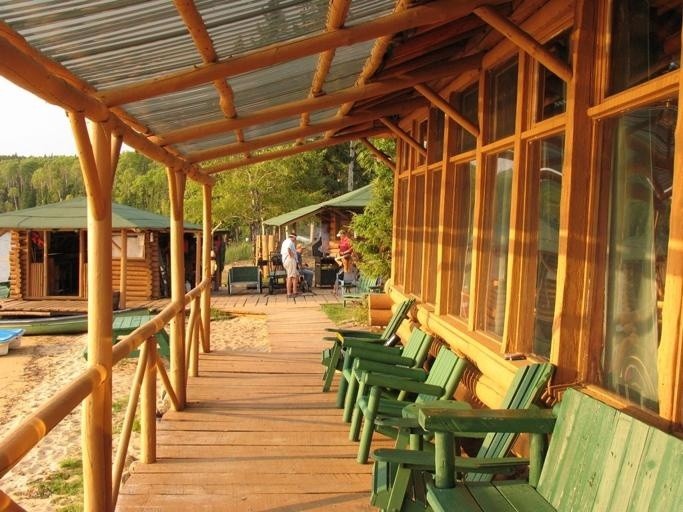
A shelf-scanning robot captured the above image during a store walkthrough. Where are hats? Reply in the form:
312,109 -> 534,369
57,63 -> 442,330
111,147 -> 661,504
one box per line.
296,243 -> 306,249
337,230 -> 346,237
289,233 -> 297,237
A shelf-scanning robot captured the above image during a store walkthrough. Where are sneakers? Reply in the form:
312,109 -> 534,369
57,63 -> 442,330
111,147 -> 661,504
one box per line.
287,292 -> 302,297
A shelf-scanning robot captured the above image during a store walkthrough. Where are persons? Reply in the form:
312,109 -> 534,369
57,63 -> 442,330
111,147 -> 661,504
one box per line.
280,233 -> 302,297
337,229 -> 353,272
296,243 -> 313,292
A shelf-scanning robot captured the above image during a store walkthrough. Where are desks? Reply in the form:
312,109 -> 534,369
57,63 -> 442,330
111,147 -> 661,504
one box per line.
83,316 -> 169,357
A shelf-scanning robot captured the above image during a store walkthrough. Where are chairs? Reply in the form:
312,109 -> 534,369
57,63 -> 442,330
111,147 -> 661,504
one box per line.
268,251 -> 304,293
343,272 -> 379,307
227,266 -> 262,295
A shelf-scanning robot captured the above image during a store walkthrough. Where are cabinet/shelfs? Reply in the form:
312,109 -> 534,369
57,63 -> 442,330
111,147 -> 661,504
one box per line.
315,256 -> 335,286
